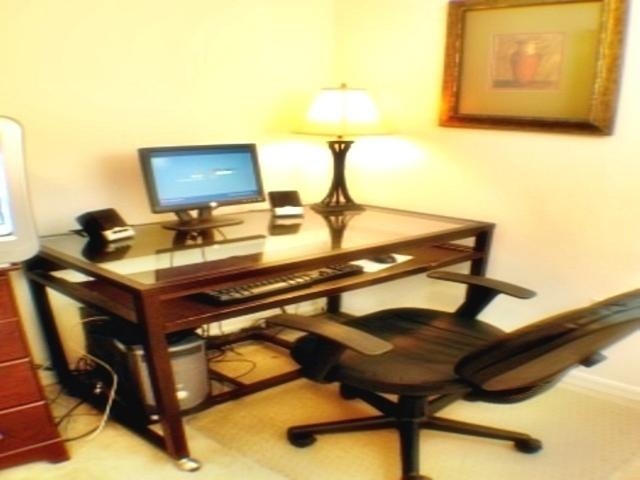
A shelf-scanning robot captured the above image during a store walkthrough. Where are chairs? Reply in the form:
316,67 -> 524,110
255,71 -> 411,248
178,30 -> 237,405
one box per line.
266,271 -> 640,480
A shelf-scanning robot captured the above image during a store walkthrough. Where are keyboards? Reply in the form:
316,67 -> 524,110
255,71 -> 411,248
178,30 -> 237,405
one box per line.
184,263 -> 363,305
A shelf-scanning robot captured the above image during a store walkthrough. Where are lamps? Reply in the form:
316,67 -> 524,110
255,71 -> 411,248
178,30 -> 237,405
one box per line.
295,82 -> 400,211
316,211 -> 363,249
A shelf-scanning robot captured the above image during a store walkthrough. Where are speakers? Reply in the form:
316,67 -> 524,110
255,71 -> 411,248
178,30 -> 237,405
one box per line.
76,208 -> 136,243
268,214 -> 304,236
81,239 -> 131,264
268,190 -> 304,216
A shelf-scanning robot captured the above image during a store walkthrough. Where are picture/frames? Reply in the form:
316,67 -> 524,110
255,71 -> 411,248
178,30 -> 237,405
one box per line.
438,0 -> 631,136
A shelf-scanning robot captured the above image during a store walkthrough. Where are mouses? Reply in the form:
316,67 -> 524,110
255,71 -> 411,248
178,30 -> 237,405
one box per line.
367,254 -> 396,263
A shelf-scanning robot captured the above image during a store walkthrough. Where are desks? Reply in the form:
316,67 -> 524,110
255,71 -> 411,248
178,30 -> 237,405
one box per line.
23,203 -> 496,462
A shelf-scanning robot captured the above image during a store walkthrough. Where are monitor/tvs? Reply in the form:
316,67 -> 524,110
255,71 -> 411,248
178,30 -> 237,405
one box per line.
0,115 -> 40,267
137,144 -> 266,231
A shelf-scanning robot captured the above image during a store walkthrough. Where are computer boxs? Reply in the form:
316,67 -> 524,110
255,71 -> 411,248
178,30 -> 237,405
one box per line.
79,307 -> 210,426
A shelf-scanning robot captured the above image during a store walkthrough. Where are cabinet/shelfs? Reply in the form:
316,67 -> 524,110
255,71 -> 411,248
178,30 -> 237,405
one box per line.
1,263 -> 69,470
